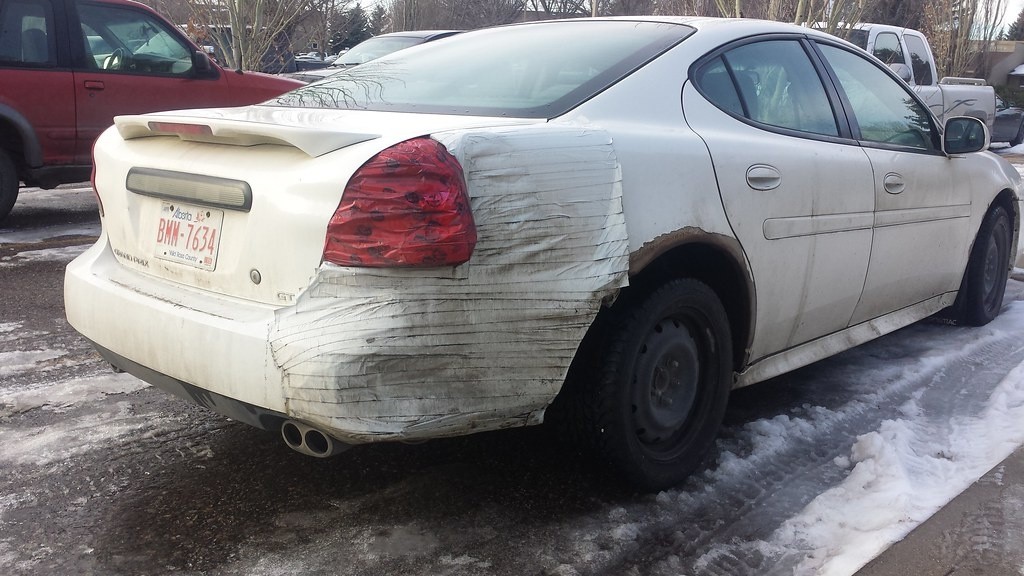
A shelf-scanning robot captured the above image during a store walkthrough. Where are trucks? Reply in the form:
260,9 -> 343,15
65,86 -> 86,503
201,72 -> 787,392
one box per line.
811,21 -> 995,145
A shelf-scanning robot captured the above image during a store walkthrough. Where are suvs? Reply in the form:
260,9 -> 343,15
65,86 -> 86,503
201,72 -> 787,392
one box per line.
0,0 -> 312,226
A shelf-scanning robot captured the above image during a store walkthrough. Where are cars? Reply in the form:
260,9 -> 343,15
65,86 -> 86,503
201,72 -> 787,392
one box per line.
293,30 -> 466,80
295,49 -> 348,71
60,17 -> 1024,483
987,92 -> 1024,147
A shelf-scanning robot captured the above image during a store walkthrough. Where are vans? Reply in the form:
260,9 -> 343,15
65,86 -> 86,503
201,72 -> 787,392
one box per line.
179,26 -> 297,73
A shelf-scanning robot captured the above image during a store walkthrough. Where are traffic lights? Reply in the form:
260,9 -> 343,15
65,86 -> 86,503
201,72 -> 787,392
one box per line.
312,42 -> 318,48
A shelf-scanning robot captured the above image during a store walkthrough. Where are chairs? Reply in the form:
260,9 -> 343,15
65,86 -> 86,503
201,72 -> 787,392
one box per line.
21,28 -> 50,65
703,70 -> 758,122
